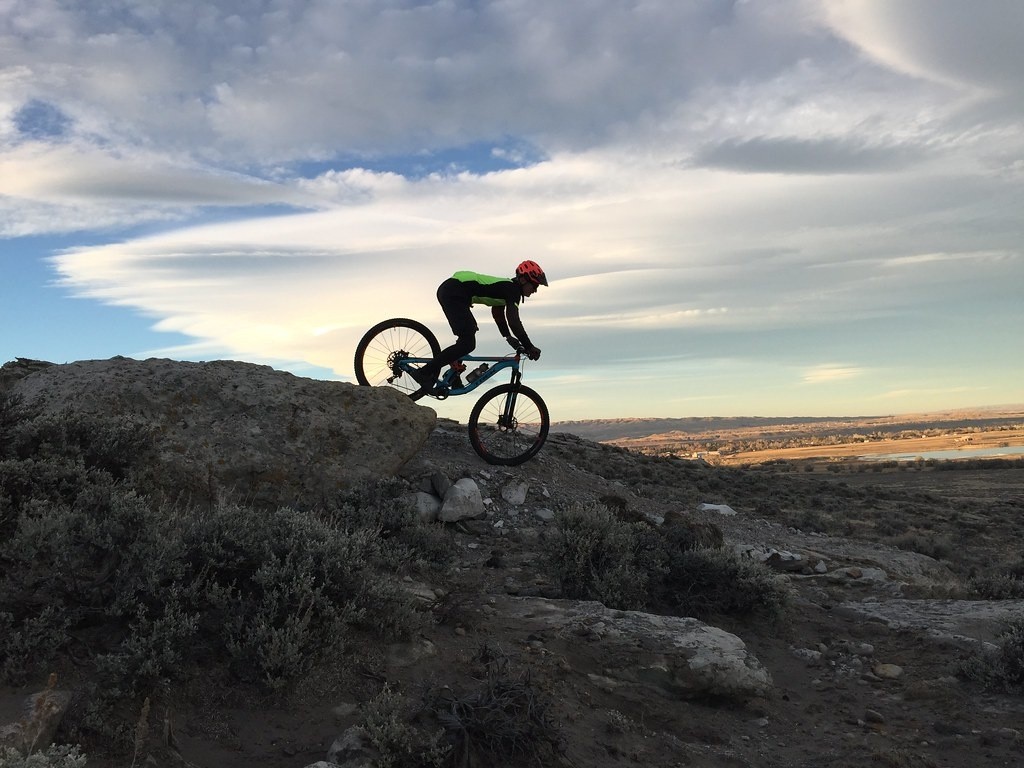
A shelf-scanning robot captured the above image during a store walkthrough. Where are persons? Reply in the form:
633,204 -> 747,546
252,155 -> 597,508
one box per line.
412,261 -> 548,393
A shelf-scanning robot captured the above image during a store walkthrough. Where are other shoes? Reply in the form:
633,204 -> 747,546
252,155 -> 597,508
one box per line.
412,364 -> 434,394
442,368 -> 465,389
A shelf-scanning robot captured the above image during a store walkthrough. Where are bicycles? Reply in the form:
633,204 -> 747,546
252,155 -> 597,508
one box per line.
351,317 -> 549,464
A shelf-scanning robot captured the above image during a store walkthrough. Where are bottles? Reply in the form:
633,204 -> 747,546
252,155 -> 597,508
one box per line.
466,363 -> 489,383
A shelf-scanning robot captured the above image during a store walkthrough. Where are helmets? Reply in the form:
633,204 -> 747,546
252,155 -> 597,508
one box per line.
516,260 -> 548,287
